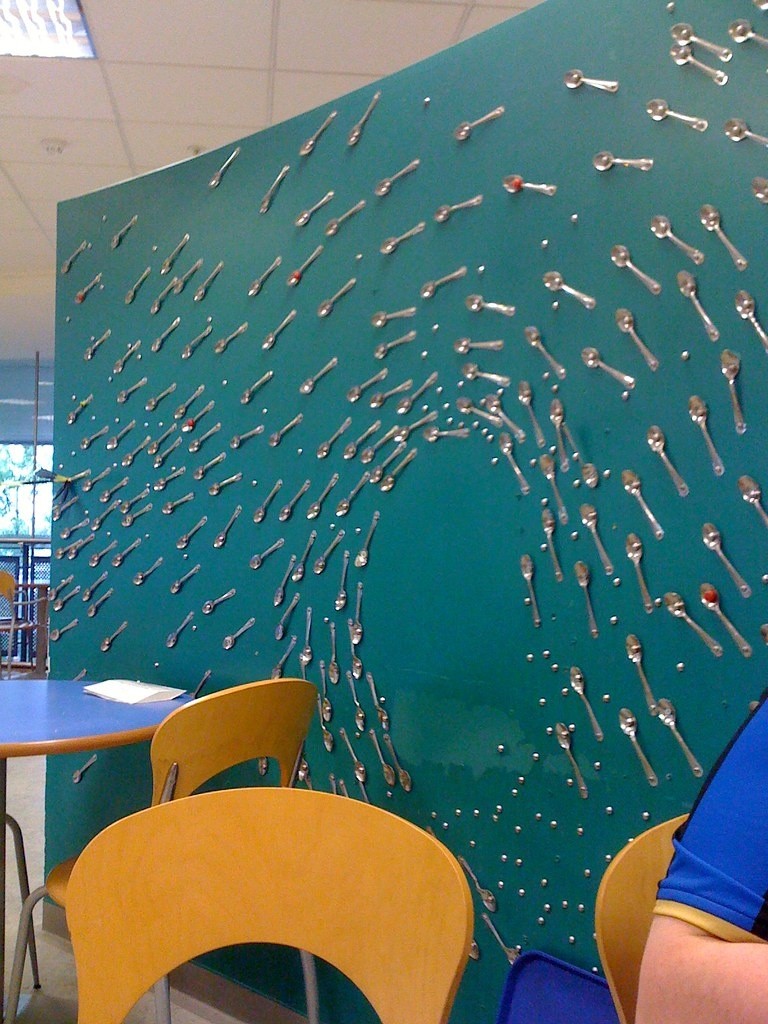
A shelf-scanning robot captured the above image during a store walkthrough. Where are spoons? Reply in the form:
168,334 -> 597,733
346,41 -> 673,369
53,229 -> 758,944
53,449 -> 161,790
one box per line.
222,616 -> 255,650
176,516 -> 208,549
668,23 -> 732,87
169,562 -> 200,593
364,671 -> 390,731
208,147 -> 241,189
132,556 -> 163,586
89,539 -> 118,568
318,660 -> 333,723
345,669 -> 366,731
728,18 -> 768,49
569,665 -> 604,741
249,70 -> 768,680
55,539 -> 83,560
456,840 -> 522,964
49,571 -> 127,652
202,587 -> 235,614
72,754 -> 97,784
165,611 -> 195,649
54,376 -> 244,539
240,369 -> 275,405
110,538 -> 142,567
67,532 -> 97,560
625,634 -> 658,716
229,425 -> 265,450
213,505 -> 242,548
556,699 -> 704,798
317,693 -> 412,793
61,215 -> 248,374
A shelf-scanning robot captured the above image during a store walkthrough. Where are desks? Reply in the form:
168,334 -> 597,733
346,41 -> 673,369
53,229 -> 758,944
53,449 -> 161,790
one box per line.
0,679 -> 193,1024
12,580 -> 50,680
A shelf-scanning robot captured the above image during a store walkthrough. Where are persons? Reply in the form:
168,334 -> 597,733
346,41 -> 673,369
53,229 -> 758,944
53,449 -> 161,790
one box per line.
630,692 -> 767,1024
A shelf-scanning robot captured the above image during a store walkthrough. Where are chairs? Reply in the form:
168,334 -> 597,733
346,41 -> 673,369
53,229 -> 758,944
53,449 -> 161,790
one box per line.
595,812 -> 690,1024
0,571 -> 36,679
0,678 -> 319,1023
66,785 -> 477,1024
